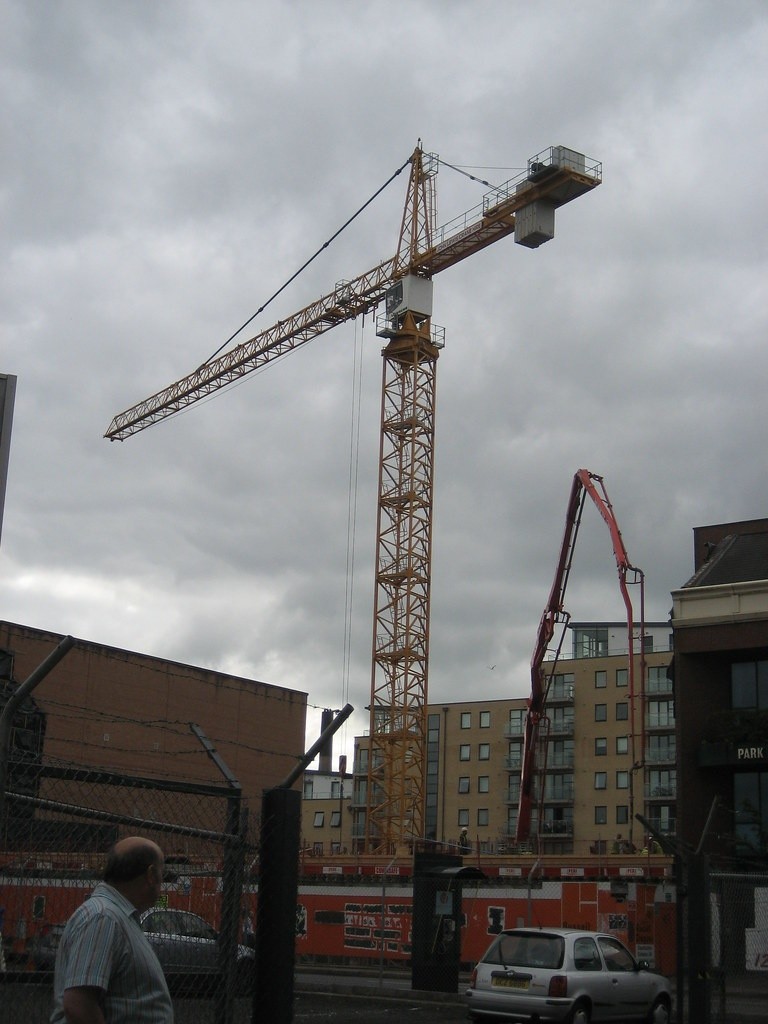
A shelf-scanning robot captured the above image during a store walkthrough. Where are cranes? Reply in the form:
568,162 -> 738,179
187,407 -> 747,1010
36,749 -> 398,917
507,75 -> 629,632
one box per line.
97,138 -> 605,837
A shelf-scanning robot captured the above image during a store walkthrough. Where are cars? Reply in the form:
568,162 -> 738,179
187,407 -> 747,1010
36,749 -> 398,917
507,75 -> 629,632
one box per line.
33,906 -> 256,997
464,925 -> 676,1024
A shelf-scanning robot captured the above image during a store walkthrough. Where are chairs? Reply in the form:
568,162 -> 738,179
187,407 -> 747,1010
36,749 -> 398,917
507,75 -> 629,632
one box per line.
575,946 -> 594,962
531,944 -> 549,958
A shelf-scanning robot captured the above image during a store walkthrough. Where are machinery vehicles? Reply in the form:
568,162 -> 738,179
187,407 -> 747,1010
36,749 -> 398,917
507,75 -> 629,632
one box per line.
510,465 -> 654,852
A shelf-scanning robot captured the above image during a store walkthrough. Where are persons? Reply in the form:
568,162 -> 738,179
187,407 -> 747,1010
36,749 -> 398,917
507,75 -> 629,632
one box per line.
609,833 -> 673,856
242,911 -> 256,949
49,837 -> 174,1024
459,827 -> 469,855
